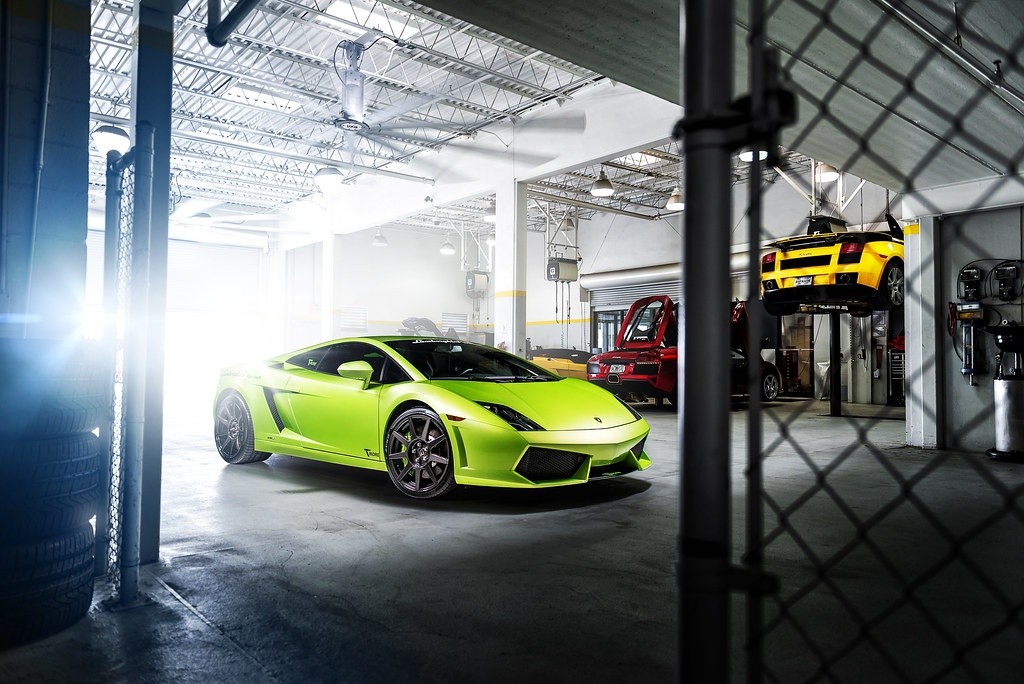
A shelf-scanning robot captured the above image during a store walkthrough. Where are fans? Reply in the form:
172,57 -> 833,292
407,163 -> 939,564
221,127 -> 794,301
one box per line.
236,43 -> 586,184
88,173 -> 318,252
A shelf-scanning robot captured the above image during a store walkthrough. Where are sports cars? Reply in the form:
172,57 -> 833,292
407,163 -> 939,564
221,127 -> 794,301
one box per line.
585,294 -> 784,405
759,215 -> 903,320
213,333 -> 655,505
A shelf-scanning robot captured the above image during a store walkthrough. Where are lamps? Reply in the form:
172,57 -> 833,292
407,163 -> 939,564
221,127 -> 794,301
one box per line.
311,151 -> 344,183
483,201 -> 496,222
485,224 -> 496,246
560,219 -> 575,231
440,233 -> 456,255
590,165 -> 614,197
371,228 -> 388,248
815,161 -> 839,183
91,104 -> 131,155
666,183 -> 684,211
739,147 -> 767,163
